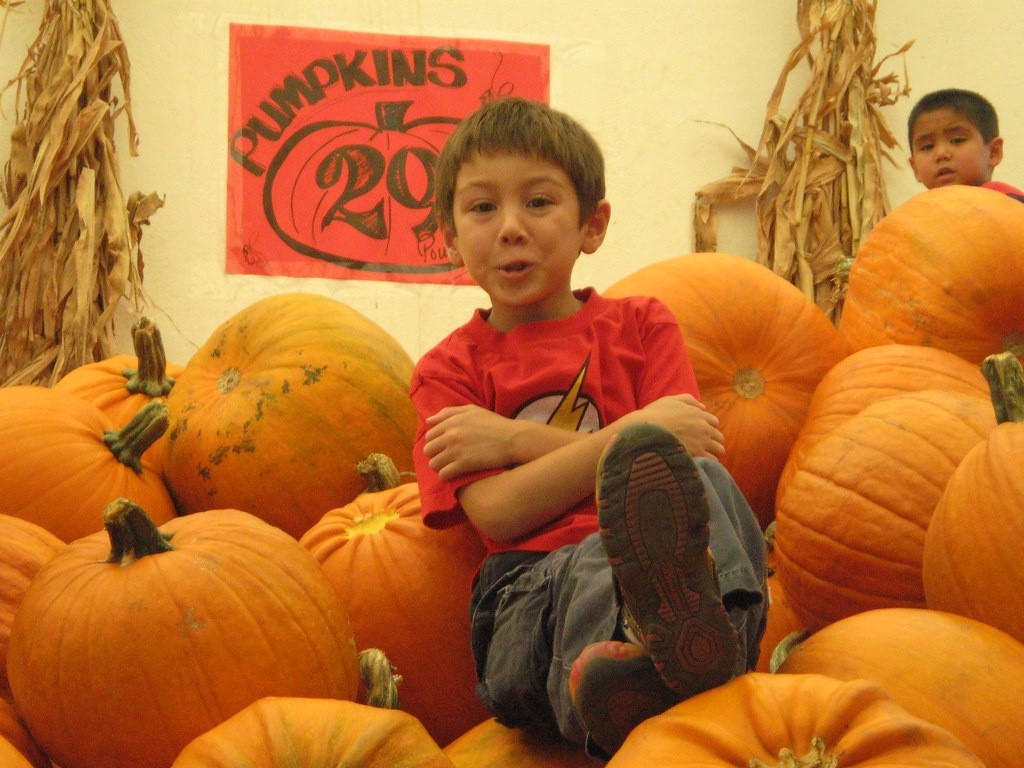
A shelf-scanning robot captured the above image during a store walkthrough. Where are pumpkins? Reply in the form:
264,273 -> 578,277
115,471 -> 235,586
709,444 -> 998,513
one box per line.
3,186 -> 1024,768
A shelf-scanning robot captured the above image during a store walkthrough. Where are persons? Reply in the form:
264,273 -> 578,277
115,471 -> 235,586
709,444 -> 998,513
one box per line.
908,87 -> 1024,204
406,95 -> 770,763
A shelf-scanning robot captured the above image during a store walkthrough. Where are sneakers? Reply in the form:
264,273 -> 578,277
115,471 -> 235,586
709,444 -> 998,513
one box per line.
571,419 -> 739,760
